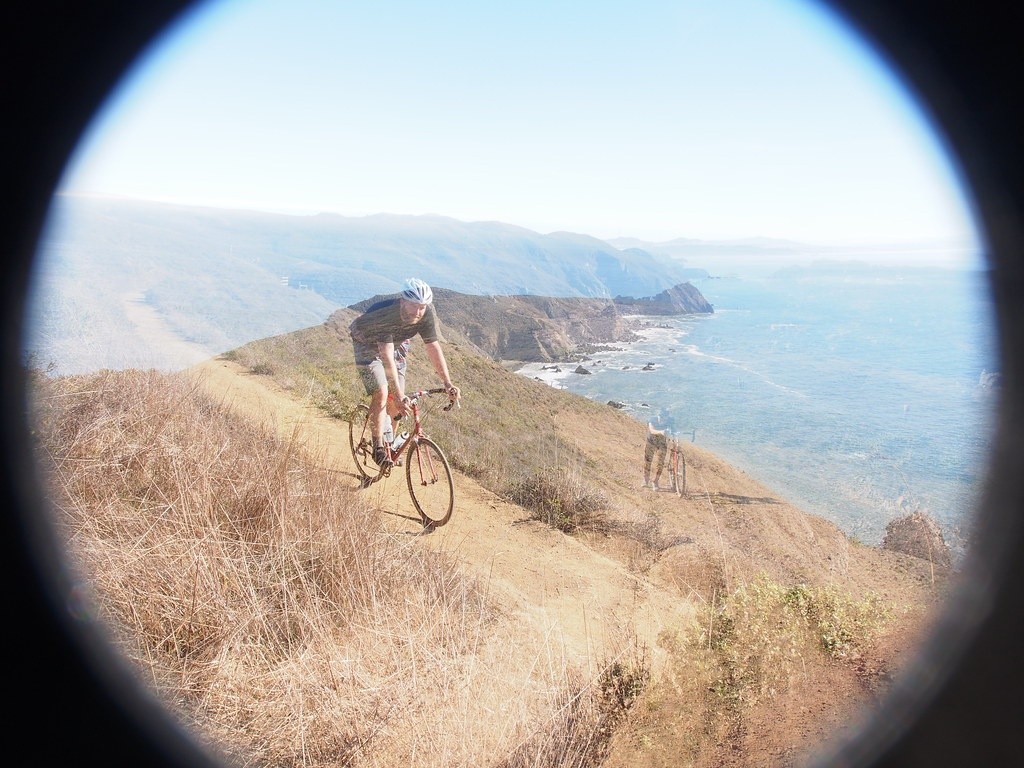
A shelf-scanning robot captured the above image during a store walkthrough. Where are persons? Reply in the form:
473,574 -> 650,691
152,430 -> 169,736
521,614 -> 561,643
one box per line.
643,409 -> 675,491
348,278 -> 460,466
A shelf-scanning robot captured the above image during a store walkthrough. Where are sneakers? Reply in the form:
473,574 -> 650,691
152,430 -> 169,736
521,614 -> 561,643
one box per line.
371,447 -> 393,468
384,443 -> 402,466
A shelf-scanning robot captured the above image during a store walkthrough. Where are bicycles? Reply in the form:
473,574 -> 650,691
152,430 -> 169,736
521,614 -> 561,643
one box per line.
348,388 -> 454,530
667,430 -> 695,496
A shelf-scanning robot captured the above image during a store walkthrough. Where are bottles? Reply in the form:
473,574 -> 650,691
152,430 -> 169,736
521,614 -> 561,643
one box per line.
383,415 -> 394,443
390,431 -> 410,451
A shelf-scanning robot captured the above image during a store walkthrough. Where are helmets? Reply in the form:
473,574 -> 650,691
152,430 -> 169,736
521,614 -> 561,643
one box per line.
400,277 -> 432,305
